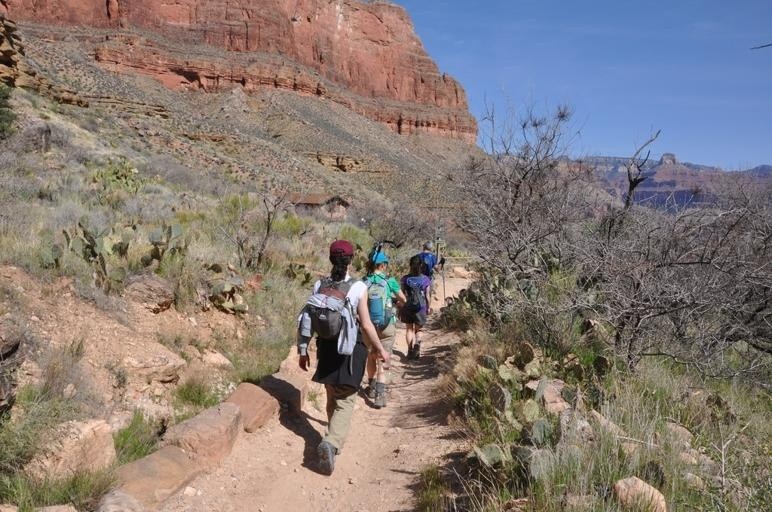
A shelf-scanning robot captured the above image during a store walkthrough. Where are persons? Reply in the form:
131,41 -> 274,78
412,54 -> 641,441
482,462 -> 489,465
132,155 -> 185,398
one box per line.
361,249 -> 407,407
417,240 -> 447,315
400,255 -> 430,362
297,240 -> 390,475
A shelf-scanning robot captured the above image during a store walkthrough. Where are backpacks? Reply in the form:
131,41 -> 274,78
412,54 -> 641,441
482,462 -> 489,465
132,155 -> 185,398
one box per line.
403,274 -> 424,314
361,274 -> 390,329
308,276 -> 357,340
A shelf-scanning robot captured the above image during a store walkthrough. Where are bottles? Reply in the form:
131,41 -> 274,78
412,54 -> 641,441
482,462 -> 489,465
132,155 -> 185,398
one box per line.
344,297 -> 353,316
386,299 -> 392,308
370,293 -> 380,299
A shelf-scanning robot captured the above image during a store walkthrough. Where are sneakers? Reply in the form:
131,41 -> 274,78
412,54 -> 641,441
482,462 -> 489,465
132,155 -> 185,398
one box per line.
317,442 -> 336,476
408,342 -> 420,361
363,386 -> 386,409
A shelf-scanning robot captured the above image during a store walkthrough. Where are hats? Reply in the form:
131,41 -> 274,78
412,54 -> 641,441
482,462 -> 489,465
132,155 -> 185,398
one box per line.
425,241 -> 433,250
330,240 -> 354,256
372,251 -> 390,264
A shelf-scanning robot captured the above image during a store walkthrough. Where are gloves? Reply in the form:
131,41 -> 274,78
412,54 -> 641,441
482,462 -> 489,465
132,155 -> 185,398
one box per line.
438,258 -> 445,268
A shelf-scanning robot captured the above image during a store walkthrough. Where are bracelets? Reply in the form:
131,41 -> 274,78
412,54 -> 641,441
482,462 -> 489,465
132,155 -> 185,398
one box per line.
439,257 -> 445,265
